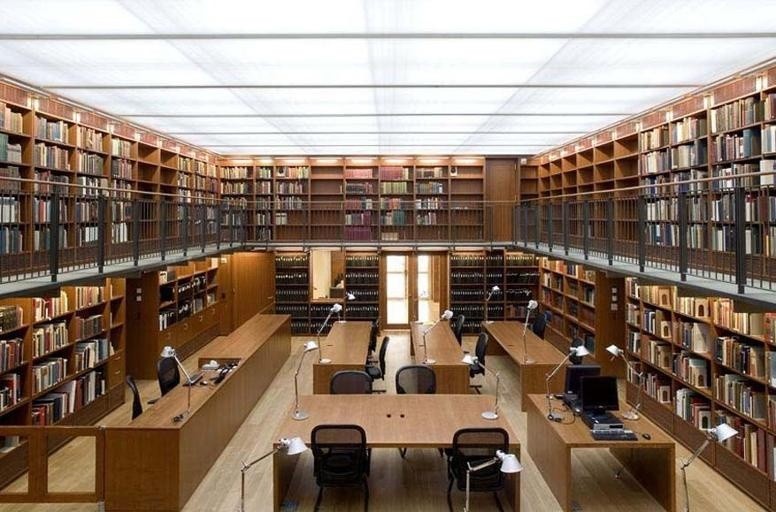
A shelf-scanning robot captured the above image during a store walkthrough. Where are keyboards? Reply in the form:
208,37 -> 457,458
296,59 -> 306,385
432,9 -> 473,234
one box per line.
554,395 -> 565,399
182,371 -> 205,386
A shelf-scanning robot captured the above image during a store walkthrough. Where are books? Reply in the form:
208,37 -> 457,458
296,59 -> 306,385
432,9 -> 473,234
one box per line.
640,91 -> 776,258
343,166 -> 445,244
535,256 -> 595,353
158,151 -> 215,332
625,277 -> 776,472
217,165 -> 308,240
0,283 -> 114,450
0,102 -> 133,252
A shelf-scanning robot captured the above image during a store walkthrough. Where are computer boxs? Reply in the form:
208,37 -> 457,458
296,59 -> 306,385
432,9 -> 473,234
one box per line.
563,393 -> 579,407
580,411 -> 625,430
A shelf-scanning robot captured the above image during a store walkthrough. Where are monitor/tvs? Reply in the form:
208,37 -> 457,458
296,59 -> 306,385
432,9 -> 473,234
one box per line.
564,366 -> 601,400
209,362 -> 232,384
580,375 -> 624,415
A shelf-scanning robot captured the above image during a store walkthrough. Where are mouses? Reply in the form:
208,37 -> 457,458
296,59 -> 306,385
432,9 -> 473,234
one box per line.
643,433 -> 650,439
200,381 -> 208,385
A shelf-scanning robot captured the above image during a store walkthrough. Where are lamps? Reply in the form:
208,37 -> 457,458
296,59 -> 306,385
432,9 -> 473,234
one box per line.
239,436 -> 308,511
